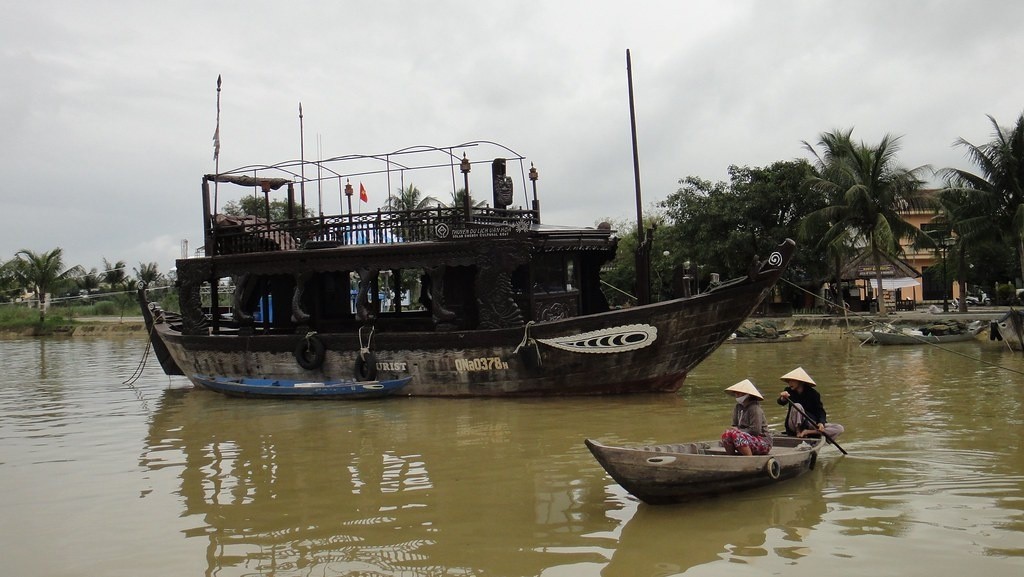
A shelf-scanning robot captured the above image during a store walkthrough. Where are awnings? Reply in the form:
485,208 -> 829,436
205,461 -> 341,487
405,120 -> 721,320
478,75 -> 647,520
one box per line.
870,277 -> 920,289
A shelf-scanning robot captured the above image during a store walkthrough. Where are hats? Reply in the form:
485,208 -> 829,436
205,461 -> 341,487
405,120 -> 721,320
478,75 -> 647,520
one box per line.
724,378 -> 765,401
780,366 -> 816,387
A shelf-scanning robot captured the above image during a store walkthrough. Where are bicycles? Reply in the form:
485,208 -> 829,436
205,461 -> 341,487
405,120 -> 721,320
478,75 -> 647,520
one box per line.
820,297 -> 850,314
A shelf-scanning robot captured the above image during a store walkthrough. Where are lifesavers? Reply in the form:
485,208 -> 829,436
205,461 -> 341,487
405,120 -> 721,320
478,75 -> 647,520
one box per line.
354,353 -> 377,382
294,336 -> 326,370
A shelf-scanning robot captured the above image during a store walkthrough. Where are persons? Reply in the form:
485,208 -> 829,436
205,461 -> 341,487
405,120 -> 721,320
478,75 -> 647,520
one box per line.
777,366 -> 844,443
975,288 -> 982,302
721,379 -> 773,455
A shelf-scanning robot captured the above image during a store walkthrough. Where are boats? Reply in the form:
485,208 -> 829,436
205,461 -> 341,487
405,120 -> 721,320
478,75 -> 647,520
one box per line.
138,139 -> 794,399
586,421 -> 845,503
853,319 -> 982,342
871,325 -> 990,345
724,328 -> 813,341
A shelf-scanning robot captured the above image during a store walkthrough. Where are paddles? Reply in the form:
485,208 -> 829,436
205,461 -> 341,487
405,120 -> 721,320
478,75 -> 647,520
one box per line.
847,323 -> 877,334
859,319 -> 897,347
782,395 -> 848,455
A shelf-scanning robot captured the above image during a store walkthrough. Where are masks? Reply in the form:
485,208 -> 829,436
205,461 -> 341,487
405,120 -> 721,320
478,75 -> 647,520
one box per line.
734,394 -> 750,406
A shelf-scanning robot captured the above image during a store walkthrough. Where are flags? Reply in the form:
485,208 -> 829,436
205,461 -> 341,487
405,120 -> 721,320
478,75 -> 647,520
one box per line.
360,184 -> 367,203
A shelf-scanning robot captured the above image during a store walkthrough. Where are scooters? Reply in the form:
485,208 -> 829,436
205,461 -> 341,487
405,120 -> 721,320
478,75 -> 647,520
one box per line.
951,288 -> 992,310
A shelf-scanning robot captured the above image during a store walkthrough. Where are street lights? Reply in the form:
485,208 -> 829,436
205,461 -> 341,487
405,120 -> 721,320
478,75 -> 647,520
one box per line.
905,244 -> 916,305
